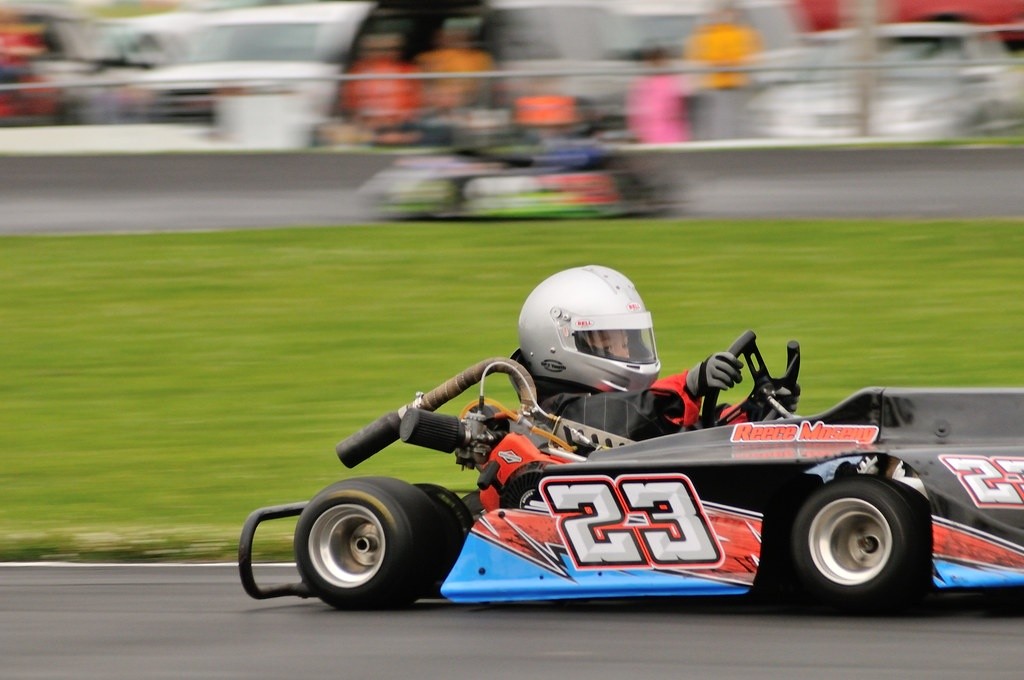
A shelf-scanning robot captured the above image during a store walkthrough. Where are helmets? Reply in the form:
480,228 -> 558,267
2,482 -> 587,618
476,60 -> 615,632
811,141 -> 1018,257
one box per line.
518,264 -> 662,394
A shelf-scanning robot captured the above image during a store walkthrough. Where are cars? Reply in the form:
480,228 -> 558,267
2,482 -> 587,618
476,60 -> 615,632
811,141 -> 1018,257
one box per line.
78,1 -> 1023,140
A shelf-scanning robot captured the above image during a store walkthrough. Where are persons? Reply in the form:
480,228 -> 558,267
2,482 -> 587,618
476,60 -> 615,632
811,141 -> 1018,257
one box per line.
617,6 -> 764,145
341,14 -> 497,120
0,8 -> 209,125
516,264 -> 800,443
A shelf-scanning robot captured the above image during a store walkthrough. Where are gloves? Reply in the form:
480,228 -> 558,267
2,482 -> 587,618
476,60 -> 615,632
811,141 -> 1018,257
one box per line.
750,381 -> 800,421
685,352 -> 744,400
